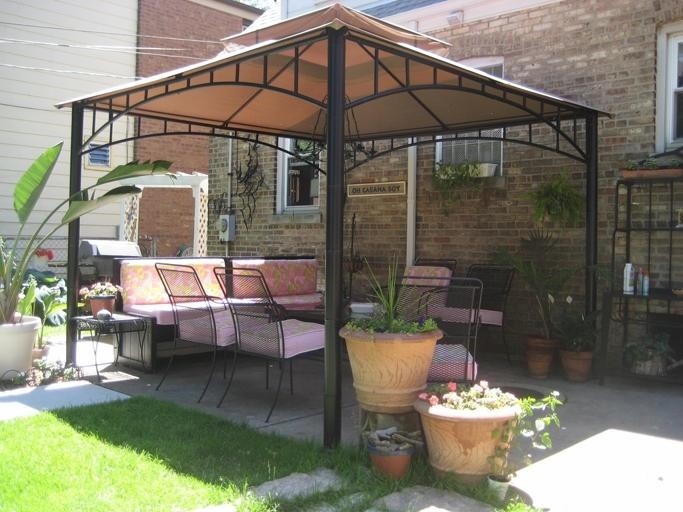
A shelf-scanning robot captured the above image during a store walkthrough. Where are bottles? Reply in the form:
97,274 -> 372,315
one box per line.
623,263 -> 649,296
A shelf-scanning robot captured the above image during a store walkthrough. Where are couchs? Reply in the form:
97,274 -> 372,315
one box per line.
113,255 -> 324,374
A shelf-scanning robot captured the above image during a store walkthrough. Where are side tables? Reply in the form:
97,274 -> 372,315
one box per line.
72,313 -> 147,380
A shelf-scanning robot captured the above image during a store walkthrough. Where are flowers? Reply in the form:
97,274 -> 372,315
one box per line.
34,248 -> 54,260
418,379 -> 517,410
487,390 -> 567,481
546,290 -> 603,352
78,281 -> 126,300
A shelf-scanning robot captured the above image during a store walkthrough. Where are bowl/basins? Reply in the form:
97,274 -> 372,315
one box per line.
672,289 -> 683,296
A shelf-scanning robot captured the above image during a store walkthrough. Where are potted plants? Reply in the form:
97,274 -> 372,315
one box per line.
28,289 -> 67,367
622,331 -> 672,376
338,246 -> 449,414
0,138 -> 177,380
488,226 -> 607,369
431,157 -> 498,217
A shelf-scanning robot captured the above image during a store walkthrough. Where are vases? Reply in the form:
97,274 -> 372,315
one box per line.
34,255 -> 49,272
366,438 -> 414,481
526,351 -> 554,379
89,295 -> 116,319
414,398 -> 522,489
487,474 -> 512,501
558,349 -> 592,383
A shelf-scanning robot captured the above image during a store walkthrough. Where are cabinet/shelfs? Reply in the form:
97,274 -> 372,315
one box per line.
599,177 -> 683,386
